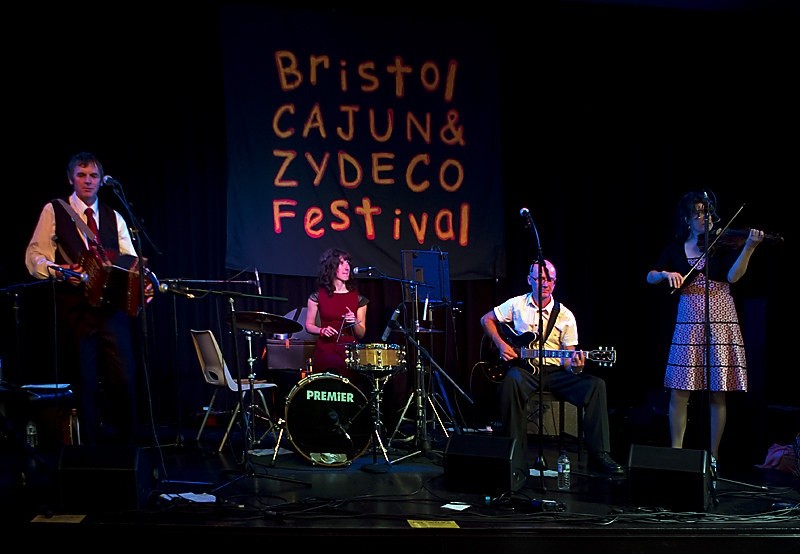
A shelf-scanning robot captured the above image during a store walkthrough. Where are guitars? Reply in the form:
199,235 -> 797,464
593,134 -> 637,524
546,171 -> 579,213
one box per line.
479,323 -> 617,385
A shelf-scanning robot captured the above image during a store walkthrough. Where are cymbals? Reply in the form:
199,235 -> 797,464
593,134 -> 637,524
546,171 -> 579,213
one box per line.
227,310 -> 304,334
389,326 -> 446,334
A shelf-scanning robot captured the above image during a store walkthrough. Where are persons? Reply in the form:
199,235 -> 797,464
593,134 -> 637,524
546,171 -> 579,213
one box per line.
24,149 -> 154,473
480,259 -> 624,476
646,192 -> 766,472
305,248 -> 369,379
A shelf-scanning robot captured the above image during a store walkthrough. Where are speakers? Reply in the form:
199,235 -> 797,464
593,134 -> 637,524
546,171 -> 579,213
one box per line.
55,447 -> 158,515
627,445 -> 713,512
444,434 -> 530,496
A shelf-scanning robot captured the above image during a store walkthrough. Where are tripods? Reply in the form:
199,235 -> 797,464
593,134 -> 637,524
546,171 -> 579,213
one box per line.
175,287 -> 313,496
529,219 -> 596,495
366,271 -> 474,467
704,202 -> 770,506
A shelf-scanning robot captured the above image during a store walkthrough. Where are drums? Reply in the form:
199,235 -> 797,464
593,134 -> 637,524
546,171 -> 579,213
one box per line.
344,342 -> 410,372
283,372 -> 376,467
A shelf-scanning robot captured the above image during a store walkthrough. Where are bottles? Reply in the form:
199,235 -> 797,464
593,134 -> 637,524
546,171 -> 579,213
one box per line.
26,420 -> 39,449
557,450 -> 571,490
69,409 -> 80,446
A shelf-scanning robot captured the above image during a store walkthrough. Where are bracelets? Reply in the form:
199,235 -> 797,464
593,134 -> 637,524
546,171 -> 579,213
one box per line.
320,327 -> 324,338
570,368 -> 582,375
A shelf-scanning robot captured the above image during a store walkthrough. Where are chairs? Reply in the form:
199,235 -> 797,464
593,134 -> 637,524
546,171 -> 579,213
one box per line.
189,329 -> 278,454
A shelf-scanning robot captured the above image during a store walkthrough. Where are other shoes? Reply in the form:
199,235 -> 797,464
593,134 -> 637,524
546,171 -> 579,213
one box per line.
513,471 -> 529,492
588,452 -> 624,474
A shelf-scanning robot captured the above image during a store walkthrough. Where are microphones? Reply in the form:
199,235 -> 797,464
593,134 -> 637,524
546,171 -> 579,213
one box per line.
703,190 -> 720,223
520,207 -> 532,229
423,294 -> 429,321
382,308 -> 400,342
103,175 -> 120,185
160,283 -> 195,299
331,416 -> 352,441
50,265 -> 89,281
254,267 -> 262,295
353,267 -> 376,274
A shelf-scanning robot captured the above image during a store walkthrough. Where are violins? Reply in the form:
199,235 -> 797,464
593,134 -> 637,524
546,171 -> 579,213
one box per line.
696,225 -> 785,253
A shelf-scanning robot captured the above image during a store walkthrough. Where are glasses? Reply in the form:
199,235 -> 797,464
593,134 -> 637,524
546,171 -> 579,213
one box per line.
530,276 -> 556,283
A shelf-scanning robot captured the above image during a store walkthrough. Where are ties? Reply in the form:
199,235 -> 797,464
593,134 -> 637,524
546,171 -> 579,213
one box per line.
84,208 -> 100,250
